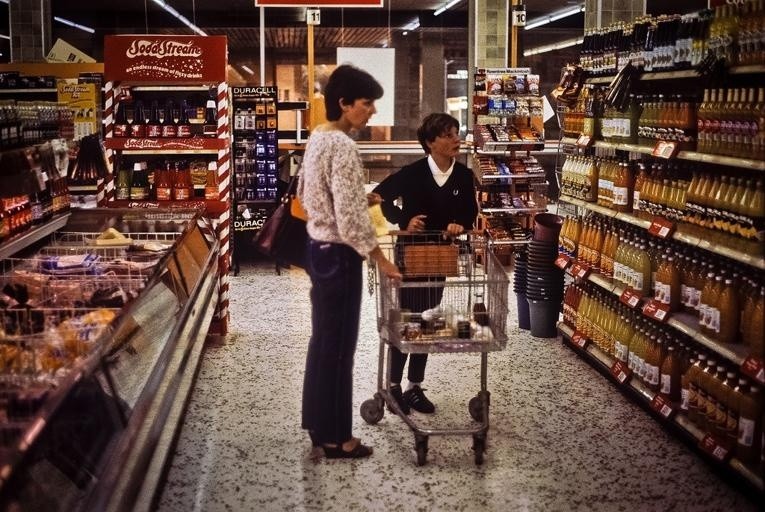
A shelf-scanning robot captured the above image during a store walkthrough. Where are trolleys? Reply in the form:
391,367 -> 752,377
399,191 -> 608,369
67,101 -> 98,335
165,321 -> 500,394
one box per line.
366,227 -> 513,468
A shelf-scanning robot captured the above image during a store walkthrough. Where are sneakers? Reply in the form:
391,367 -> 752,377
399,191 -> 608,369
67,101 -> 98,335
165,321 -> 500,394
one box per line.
403,385 -> 435,413
386,385 -> 410,415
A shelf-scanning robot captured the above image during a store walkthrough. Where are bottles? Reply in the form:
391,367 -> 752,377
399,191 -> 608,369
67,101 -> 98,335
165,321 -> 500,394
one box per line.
115,99 -> 219,201
559,0 -> 765,456
512,4 -> 526,26
472,294 -> 487,325
0,100 -> 74,244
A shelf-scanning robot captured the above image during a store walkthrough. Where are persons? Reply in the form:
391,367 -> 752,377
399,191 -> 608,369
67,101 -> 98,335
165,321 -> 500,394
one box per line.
299,63 -> 405,460
372,112 -> 480,416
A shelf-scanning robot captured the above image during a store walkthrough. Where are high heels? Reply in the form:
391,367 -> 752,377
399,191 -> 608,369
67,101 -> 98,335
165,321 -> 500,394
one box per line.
307,429 -> 373,458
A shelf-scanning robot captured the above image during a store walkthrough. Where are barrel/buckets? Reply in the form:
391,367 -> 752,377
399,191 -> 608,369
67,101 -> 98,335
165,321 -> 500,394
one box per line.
513,212 -> 564,339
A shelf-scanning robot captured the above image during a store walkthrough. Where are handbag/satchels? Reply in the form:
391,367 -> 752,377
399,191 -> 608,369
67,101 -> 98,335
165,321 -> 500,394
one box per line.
254,195 -> 306,270
